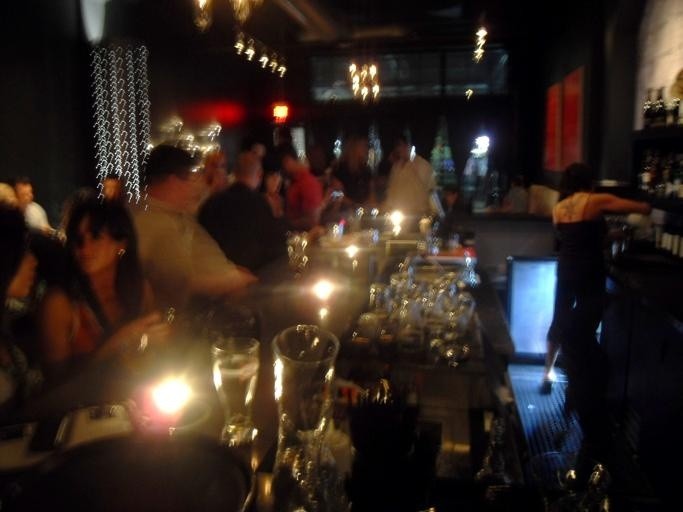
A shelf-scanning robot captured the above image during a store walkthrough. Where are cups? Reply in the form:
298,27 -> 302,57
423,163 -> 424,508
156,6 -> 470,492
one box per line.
211,324 -> 354,465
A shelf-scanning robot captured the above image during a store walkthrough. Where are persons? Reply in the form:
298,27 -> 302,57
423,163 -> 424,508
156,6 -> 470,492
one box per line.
540,163 -> 652,392
1,138 -> 478,472
501,175 -> 529,213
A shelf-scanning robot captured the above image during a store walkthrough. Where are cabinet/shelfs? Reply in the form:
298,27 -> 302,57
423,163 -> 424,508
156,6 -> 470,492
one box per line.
625,125 -> 683,213
